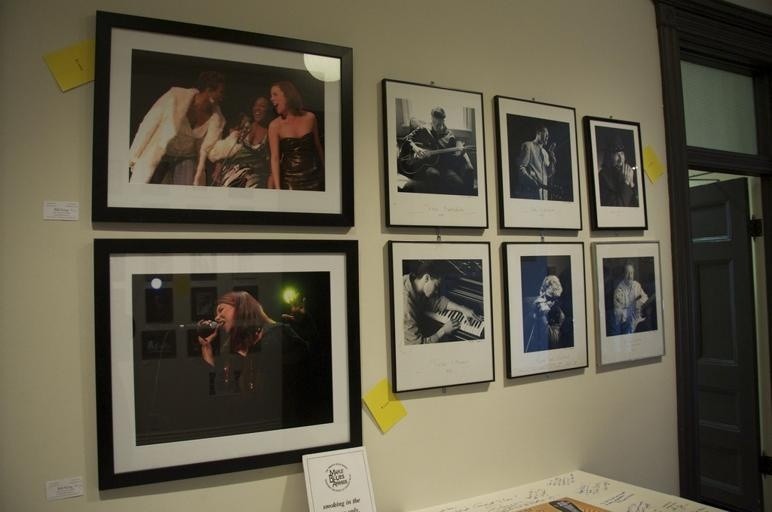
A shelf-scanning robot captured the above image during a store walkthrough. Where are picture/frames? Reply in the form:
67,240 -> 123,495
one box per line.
492,93 -> 583,232
379,76 -> 490,230
500,242 -> 590,380
580,112 -> 650,235
88,8 -> 356,229
92,235 -> 363,492
586,237 -> 667,373
387,239 -> 497,394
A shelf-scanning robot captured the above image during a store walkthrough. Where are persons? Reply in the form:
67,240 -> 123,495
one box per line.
523,274 -> 566,351
187,291 -> 317,434
404,105 -> 467,195
128,69 -> 228,189
514,123 -> 558,200
598,132 -> 638,207
211,94 -> 272,187
613,263 -> 649,336
402,261 -> 483,345
264,80 -> 324,192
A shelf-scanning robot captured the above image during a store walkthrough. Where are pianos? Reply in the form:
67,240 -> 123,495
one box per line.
419,276 -> 484,341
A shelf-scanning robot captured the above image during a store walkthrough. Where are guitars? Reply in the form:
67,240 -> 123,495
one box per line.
621,292 -> 657,334
399,140 -> 476,175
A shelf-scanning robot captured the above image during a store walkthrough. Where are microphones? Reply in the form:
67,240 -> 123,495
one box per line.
549,142 -> 557,153
178,322 -> 217,330
238,121 -> 252,141
